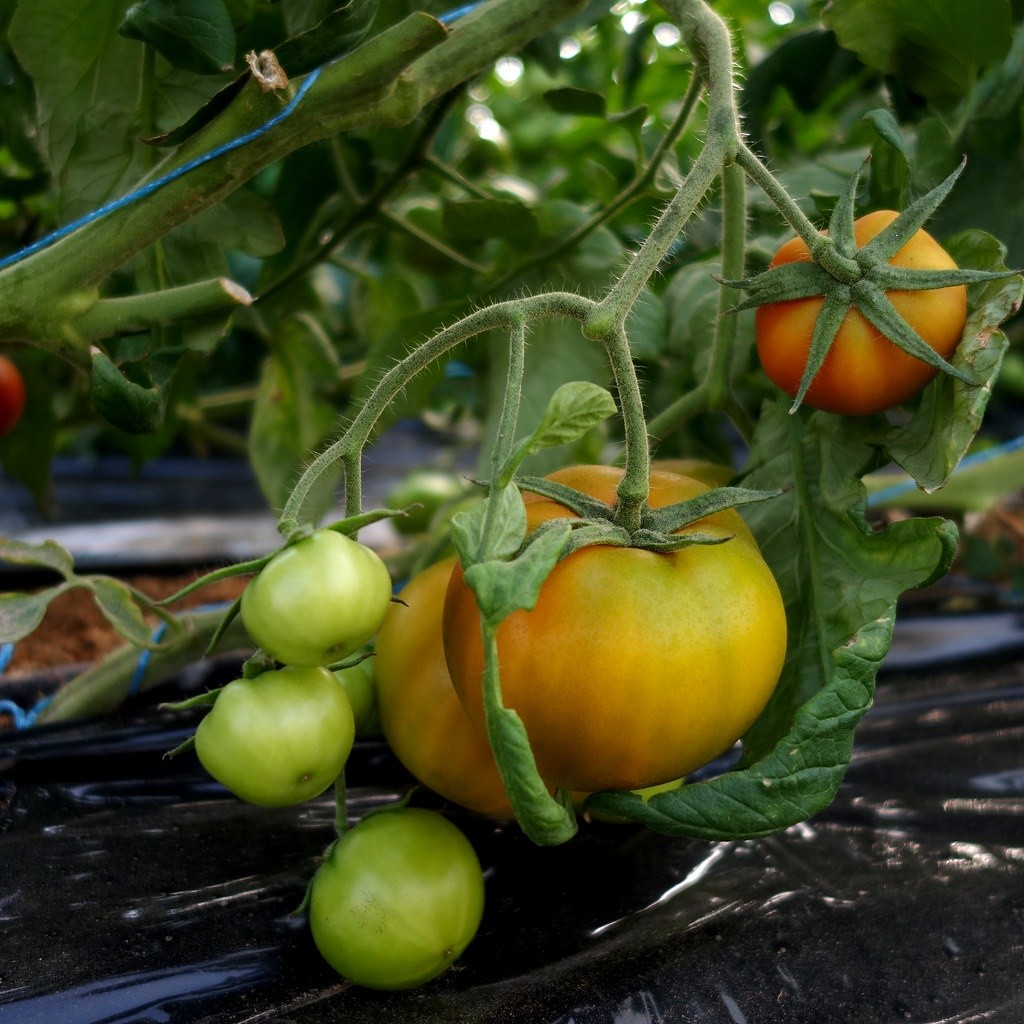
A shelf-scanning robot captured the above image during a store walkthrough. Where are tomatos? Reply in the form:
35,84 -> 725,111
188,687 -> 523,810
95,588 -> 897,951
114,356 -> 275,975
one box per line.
0,356 -> 23,433
194,464 -> 788,988
755,211 -> 969,416
